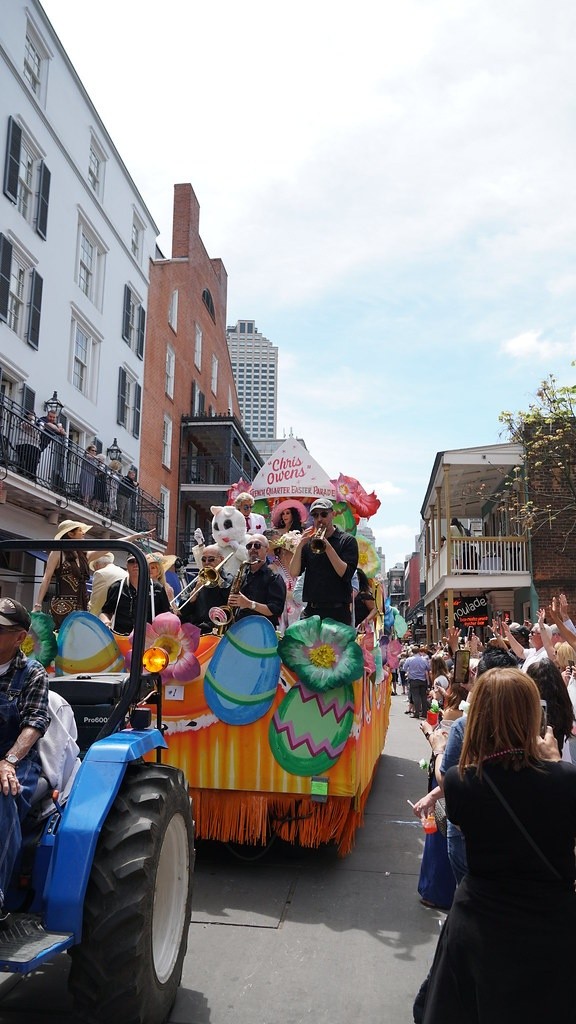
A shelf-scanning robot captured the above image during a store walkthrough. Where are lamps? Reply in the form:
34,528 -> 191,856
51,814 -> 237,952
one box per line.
107,436 -> 123,461
40,387 -> 64,418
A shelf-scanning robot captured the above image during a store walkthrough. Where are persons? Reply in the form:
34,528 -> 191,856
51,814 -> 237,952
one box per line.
234,492 -> 376,629
86,550 -> 129,616
117,469 -> 141,523
32,519 -> 157,636
414,666 -> 576,1023
99,552 -> 197,635
178,545 -> 234,633
228,533 -> 286,630
0,597 -> 53,921
440,518 -> 480,576
79,444 -> 122,518
393,579 -> 403,592
16,410 -> 67,483
390,594 -> 576,910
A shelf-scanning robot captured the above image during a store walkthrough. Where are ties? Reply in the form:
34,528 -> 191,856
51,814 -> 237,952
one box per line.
245,517 -> 251,533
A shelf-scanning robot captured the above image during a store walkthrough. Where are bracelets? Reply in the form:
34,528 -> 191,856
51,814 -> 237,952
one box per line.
34,603 -> 41,607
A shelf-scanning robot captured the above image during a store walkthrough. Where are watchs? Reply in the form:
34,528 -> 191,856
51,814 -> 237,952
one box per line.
4,754 -> 19,764
541,628 -> 545,631
424,731 -> 431,739
252,601 -> 256,610
431,751 -> 445,757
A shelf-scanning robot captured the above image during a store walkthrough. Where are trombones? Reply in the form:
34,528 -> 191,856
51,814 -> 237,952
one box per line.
169,551 -> 235,611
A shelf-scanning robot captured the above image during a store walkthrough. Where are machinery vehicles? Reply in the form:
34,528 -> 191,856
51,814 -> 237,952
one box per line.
0,540 -> 199,1024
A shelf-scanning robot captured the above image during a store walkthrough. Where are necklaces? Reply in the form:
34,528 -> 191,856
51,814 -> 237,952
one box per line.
484,748 -> 531,759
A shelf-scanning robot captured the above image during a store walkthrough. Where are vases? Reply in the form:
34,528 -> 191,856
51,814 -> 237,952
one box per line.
426,709 -> 439,725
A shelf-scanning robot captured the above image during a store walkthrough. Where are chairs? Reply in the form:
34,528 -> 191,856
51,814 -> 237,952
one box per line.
0,433 -> 20,470
19,686 -> 79,828
50,466 -> 80,497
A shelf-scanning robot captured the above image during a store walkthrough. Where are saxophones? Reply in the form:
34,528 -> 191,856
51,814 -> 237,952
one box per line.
211,557 -> 262,635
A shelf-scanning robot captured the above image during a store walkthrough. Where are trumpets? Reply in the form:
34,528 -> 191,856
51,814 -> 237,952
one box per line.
309,523 -> 328,554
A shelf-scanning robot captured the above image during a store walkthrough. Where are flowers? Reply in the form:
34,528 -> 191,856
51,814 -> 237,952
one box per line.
353,535 -> 382,578
329,471 -> 381,528
225,477 -> 252,506
275,530 -> 302,548
127,609 -> 203,685
429,697 -> 440,711
418,756 -> 429,772
21,606 -> 57,666
361,632 -> 402,692
277,615 -> 365,695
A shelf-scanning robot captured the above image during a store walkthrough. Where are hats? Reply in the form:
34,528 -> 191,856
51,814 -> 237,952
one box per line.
162,552 -> 177,574
53,520 -> 93,540
0,596 -> 32,631
272,500 -> 309,527
267,529 -> 303,554
509,625 -> 530,639
145,553 -> 167,564
310,497 -> 333,514
89,552 -> 114,571
126,552 -> 136,562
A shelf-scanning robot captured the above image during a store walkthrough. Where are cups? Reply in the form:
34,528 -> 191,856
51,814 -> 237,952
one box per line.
440,720 -> 455,740
419,806 -> 438,834
427,711 -> 438,725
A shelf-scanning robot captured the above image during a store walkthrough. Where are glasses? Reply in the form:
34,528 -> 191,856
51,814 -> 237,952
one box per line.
127,559 -> 138,564
311,509 -> 333,518
246,543 -> 268,550
529,630 -> 541,636
201,555 -> 221,563
239,505 -> 253,510
0,627 -> 24,635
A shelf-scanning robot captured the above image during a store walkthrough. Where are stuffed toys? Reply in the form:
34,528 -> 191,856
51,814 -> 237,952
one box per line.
192,505 -> 251,578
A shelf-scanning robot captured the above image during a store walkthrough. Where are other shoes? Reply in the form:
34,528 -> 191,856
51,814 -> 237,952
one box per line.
420,898 -> 437,908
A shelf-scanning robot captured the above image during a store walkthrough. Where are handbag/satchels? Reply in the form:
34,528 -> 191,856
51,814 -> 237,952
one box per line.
434,798 -> 448,837
